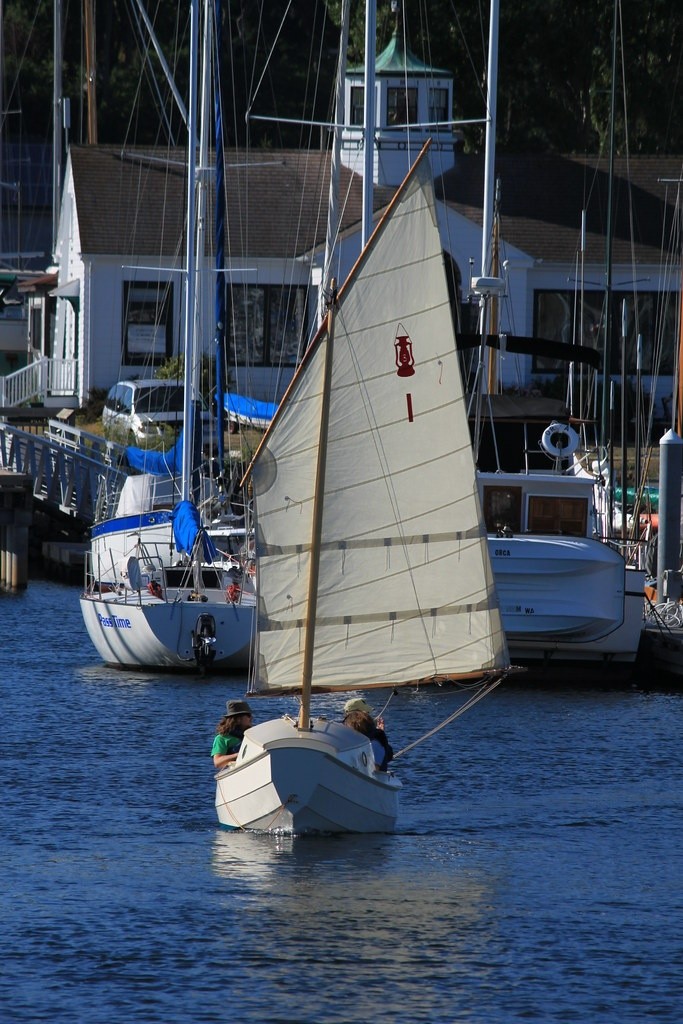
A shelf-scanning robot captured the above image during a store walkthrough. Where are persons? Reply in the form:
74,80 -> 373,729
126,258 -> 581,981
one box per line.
211,699 -> 256,769
342,698 -> 394,773
147,577 -> 166,601
226,581 -> 243,604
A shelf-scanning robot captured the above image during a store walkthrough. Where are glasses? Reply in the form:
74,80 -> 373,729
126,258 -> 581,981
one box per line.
244,713 -> 252,718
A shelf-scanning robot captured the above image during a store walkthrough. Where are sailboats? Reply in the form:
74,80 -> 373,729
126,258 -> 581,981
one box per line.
209,138 -> 529,836
0,2 -> 682,675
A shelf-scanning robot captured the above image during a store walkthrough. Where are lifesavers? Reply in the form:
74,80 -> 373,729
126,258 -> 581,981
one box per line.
543,422 -> 582,457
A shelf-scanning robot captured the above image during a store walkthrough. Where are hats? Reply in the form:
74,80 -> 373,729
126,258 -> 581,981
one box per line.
344,698 -> 375,714
221,700 -> 255,717
